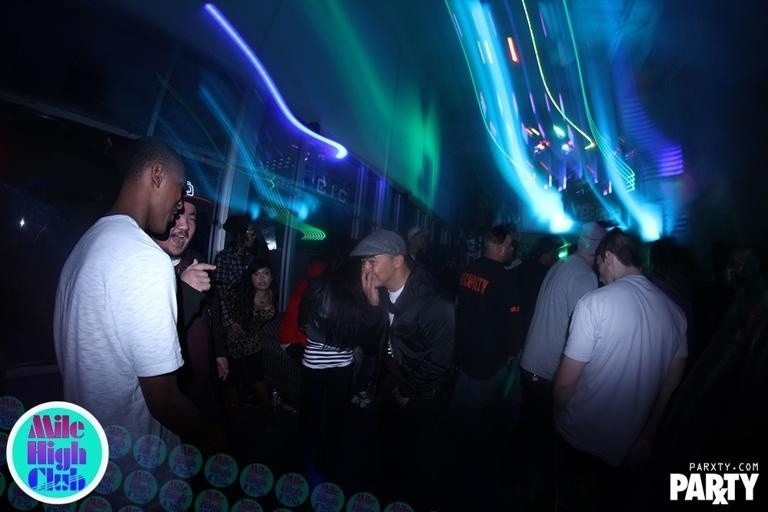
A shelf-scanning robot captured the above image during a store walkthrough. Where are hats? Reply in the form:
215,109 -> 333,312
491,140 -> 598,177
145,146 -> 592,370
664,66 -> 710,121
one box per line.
185,181 -> 213,209
407,227 -> 420,240
223,212 -> 250,244
577,222 -> 606,254
351,229 -> 407,257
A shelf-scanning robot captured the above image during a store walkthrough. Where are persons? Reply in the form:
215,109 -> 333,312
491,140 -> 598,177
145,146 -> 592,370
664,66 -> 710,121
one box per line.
263,213 -> 740,483
49,136 -> 221,511
145,178 -> 232,411
222,259 -> 279,412
214,211 -> 264,333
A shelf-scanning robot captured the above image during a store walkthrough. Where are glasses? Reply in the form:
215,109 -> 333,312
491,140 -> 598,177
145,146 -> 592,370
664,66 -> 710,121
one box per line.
245,230 -> 257,237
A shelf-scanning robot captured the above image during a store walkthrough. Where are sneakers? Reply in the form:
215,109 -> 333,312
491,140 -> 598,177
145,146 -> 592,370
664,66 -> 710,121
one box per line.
241,395 -> 261,410
272,388 -> 282,408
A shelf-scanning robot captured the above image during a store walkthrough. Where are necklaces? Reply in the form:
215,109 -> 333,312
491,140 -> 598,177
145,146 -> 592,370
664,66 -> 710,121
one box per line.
258,300 -> 265,304
